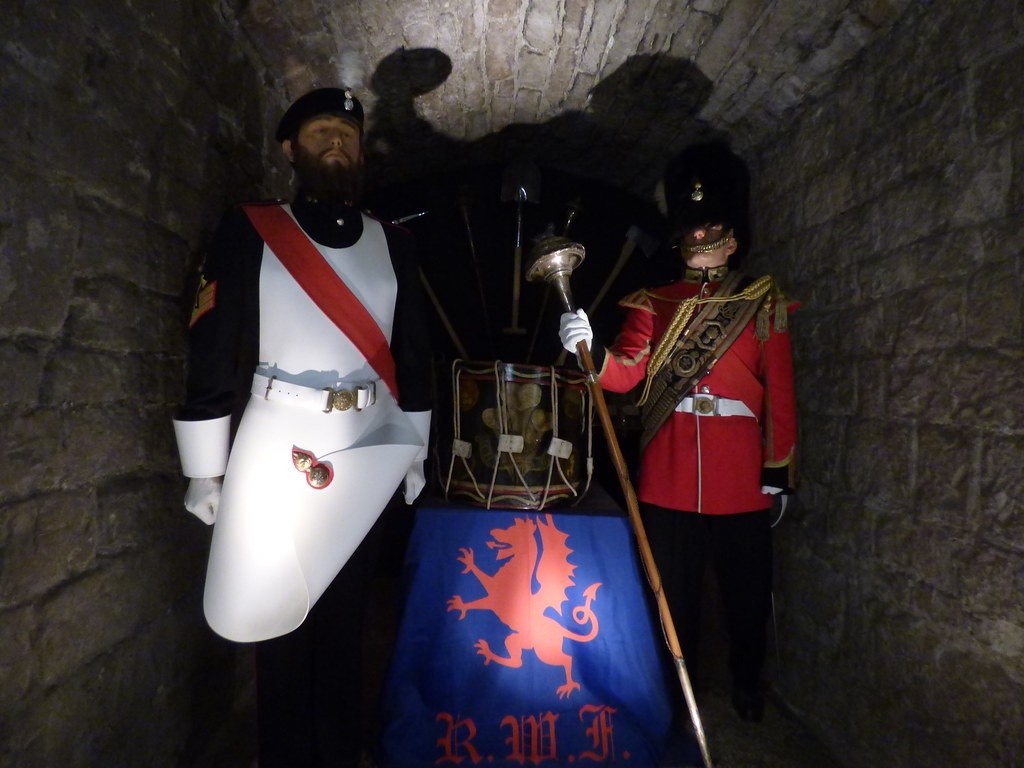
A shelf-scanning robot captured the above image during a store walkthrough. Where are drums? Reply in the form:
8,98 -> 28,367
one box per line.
431,357 -> 597,512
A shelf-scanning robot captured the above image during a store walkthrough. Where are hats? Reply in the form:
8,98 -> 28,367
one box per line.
275,88 -> 364,144
660,141 -> 749,273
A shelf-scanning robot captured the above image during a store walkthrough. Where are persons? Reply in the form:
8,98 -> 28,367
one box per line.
173,88 -> 451,768
558,190 -> 797,723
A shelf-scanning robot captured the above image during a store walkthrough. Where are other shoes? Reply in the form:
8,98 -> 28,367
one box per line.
673,689 -> 695,719
732,690 -> 764,723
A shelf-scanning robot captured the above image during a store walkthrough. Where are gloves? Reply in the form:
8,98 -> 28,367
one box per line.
403,460 -> 431,505
183,477 -> 224,525
559,308 -> 593,360
761,485 -> 784,495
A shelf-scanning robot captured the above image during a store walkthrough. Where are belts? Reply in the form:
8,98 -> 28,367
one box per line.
250,373 -> 390,412
673,393 -> 756,417
